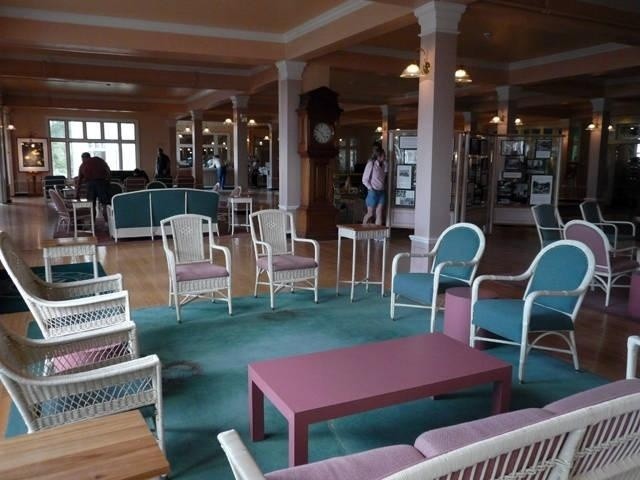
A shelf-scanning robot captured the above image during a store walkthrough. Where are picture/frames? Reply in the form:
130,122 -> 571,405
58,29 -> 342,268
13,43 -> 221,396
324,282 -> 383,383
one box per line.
385,133 -> 561,187
16,136 -> 51,174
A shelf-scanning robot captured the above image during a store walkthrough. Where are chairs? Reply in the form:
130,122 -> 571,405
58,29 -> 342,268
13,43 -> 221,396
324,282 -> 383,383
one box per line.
248,208 -> 319,307
37,172 -> 252,241
580,201 -> 635,259
391,220 -> 483,335
1,321 -> 164,456
158,214 -> 233,321
471,238 -> 596,384
0,228 -> 131,333
531,203 -> 567,248
562,219 -> 638,306
216,335 -> 640,478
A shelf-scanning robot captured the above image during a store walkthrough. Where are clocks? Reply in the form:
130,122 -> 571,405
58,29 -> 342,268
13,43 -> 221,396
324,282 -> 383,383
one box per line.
295,83 -> 347,160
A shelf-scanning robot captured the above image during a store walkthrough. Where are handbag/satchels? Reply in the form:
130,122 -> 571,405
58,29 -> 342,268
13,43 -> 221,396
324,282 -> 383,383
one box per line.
358,184 -> 368,200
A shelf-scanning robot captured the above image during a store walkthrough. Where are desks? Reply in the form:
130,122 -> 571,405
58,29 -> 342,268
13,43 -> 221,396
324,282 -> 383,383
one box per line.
42,246 -> 99,282
2,409 -> 177,476
335,222 -> 388,303
248,332 -> 513,466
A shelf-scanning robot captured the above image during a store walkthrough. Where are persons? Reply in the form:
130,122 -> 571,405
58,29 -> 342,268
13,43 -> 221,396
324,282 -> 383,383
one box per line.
361,149 -> 389,225
156,148 -> 170,175
210,155 -> 226,189
76,153 -> 102,226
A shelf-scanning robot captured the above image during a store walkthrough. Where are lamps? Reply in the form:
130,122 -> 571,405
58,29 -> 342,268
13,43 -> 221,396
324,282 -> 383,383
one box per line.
453,59 -> 473,86
399,43 -> 433,81
221,118 -> 236,126
6,110 -> 17,131
510,115 -> 525,127
486,114 -> 506,126
585,122 -> 599,131
605,125 -> 616,134
201,120 -> 213,134
246,117 -> 258,127
